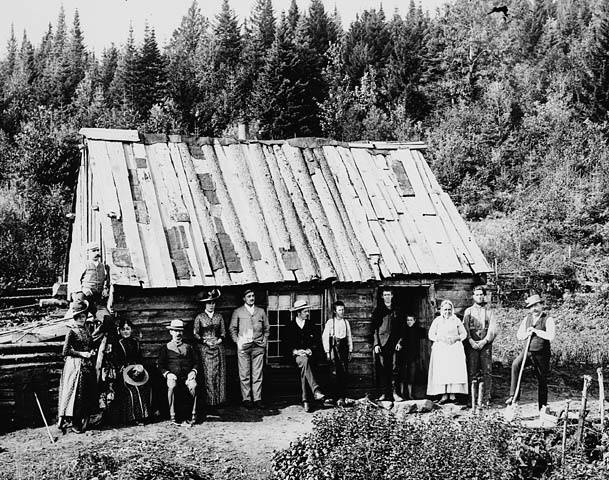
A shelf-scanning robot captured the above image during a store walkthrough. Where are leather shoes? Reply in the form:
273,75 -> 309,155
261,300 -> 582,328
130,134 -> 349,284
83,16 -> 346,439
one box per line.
314,392 -> 326,400
304,402 -> 311,412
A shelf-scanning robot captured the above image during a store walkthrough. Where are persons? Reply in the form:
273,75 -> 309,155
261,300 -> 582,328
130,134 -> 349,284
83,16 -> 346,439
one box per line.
401,312 -> 428,400
78,243 -> 114,319
157,319 -> 199,423
426,299 -> 468,405
282,299 -> 325,413
229,290 -> 270,409
113,319 -> 148,425
370,287 -> 403,402
57,298 -> 95,434
505,294 -> 555,411
194,289 -> 227,407
321,301 -> 353,372
463,287 -> 497,406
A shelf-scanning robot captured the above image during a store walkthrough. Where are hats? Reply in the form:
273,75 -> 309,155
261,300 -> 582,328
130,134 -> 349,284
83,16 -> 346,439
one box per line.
196,288 -> 222,302
122,364 -> 150,386
166,319 -> 185,330
64,298 -> 89,319
289,300 -> 310,312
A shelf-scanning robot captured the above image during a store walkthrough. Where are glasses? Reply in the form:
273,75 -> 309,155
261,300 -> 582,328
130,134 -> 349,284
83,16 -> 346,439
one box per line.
92,248 -> 100,252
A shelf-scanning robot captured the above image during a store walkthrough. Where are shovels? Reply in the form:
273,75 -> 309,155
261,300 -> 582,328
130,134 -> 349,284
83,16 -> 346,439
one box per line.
505,330 -> 534,424
